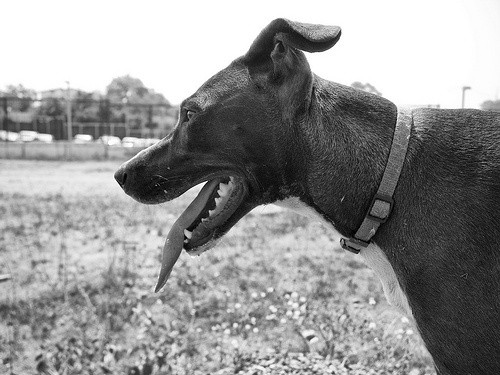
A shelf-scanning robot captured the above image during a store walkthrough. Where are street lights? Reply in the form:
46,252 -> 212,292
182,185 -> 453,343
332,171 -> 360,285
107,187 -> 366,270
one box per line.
461,85 -> 472,108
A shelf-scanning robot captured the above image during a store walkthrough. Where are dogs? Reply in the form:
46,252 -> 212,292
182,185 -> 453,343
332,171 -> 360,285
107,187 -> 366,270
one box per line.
113,17 -> 500,375
